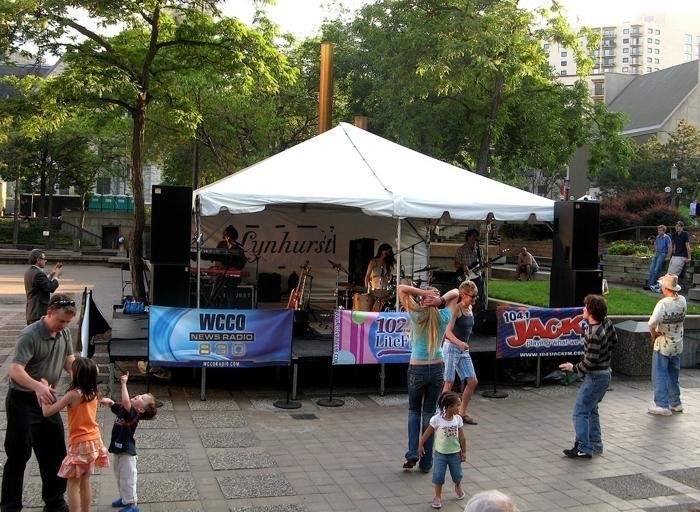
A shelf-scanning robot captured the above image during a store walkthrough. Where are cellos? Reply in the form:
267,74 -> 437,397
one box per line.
287,261 -> 310,310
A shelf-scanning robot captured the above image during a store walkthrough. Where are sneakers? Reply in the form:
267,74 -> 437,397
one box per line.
649,405 -> 672,417
112,499 -> 139,511
563,444 -> 593,459
403,456 -> 433,473
671,404 -> 684,413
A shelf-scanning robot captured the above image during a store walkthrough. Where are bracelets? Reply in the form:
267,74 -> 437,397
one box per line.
440,297 -> 445,304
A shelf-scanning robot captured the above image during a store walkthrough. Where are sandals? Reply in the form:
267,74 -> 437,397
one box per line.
462,415 -> 477,426
432,486 -> 465,508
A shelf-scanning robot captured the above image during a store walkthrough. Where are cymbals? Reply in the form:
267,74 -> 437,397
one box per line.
328,260 -> 349,275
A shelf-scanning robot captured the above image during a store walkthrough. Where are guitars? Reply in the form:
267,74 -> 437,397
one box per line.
456,249 -> 510,285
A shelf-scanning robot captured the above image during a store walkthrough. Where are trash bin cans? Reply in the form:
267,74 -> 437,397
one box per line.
101,223 -> 120,249
143,224 -> 149,260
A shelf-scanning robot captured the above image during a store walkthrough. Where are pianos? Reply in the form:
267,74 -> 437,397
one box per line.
191,267 -> 249,284
191,248 -> 241,261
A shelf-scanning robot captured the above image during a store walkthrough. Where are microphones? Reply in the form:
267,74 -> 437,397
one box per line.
228,236 -> 231,241
475,239 -> 480,243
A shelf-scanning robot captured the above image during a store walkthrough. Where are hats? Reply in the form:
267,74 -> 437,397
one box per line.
657,273 -> 682,292
675,221 -> 684,226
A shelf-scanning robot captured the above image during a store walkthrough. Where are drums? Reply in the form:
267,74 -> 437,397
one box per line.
352,293 -> 372,311
368,276 -> 391,297
374,295 -> 406,311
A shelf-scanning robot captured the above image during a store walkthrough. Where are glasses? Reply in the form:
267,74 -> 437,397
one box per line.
463,292 -> 479,298
48,301 -> 75,307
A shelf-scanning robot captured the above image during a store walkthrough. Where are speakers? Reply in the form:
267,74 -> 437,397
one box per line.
149,265 -> 191,308
151,185 -> 193,264
552,200 -> 600,270
549,269 -> 603,309
349,238 -> 376,285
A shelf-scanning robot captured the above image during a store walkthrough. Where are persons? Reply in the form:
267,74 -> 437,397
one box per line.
689,200 -> 697,218
99,370 -> 157,512
441,280 -> 479,426
39,357 -> 109,512
695,200 -> 700,218
513,246 -> 539,282
642,224 -> 673,290
207,224 -> 247,309
23,247 -> 63,327
0,293 -> 76,512
396,284 -> 460,473
647,272 -> 688,417
418,391 -> 468,509
558,294 -> 618,458
650,220 -> 692,294
364,243 -> 395,310
454,228 -> 493,316
